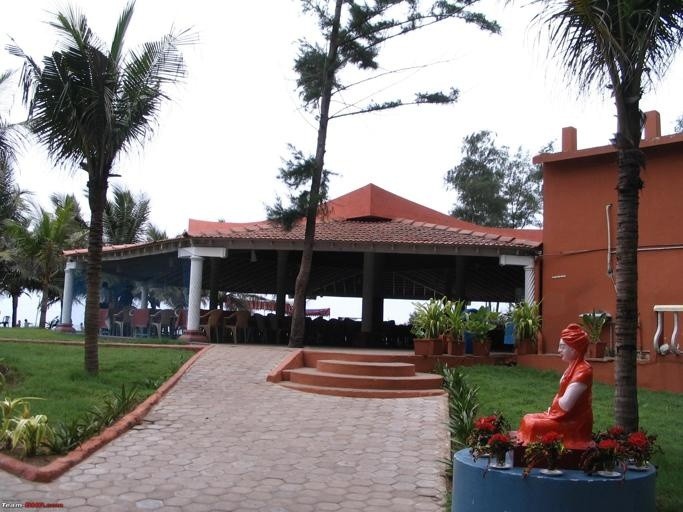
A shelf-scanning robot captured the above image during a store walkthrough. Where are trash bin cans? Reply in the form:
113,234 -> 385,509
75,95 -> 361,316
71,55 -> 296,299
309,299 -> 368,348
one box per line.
462,330 -> 480,354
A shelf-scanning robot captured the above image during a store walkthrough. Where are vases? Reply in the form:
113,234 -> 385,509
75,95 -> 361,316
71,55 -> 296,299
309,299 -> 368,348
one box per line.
598,462 -> 623,478
541,458 -> 565,475
489,455 -> 514,469
631,458 -> 651,471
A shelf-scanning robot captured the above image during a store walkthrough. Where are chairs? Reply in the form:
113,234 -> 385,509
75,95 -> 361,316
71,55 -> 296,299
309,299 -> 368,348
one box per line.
99,305 -> 405,347
0,316 -> 10,328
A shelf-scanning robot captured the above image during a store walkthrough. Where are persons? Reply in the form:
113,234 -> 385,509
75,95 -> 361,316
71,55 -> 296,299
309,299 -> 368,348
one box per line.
16,320 -> 21,328
98,280 -> 112,336
24,319 -> 32,327
117,285 -> 134,336
517,322 -> 594,440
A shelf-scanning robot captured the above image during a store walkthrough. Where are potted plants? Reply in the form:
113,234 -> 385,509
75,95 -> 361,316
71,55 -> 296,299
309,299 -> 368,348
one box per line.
582,309 -> 609,357
413,298 -> 544,355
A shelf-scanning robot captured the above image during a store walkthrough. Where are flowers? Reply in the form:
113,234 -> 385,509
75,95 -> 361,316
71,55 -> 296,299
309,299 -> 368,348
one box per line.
473,416 -> 659,457
